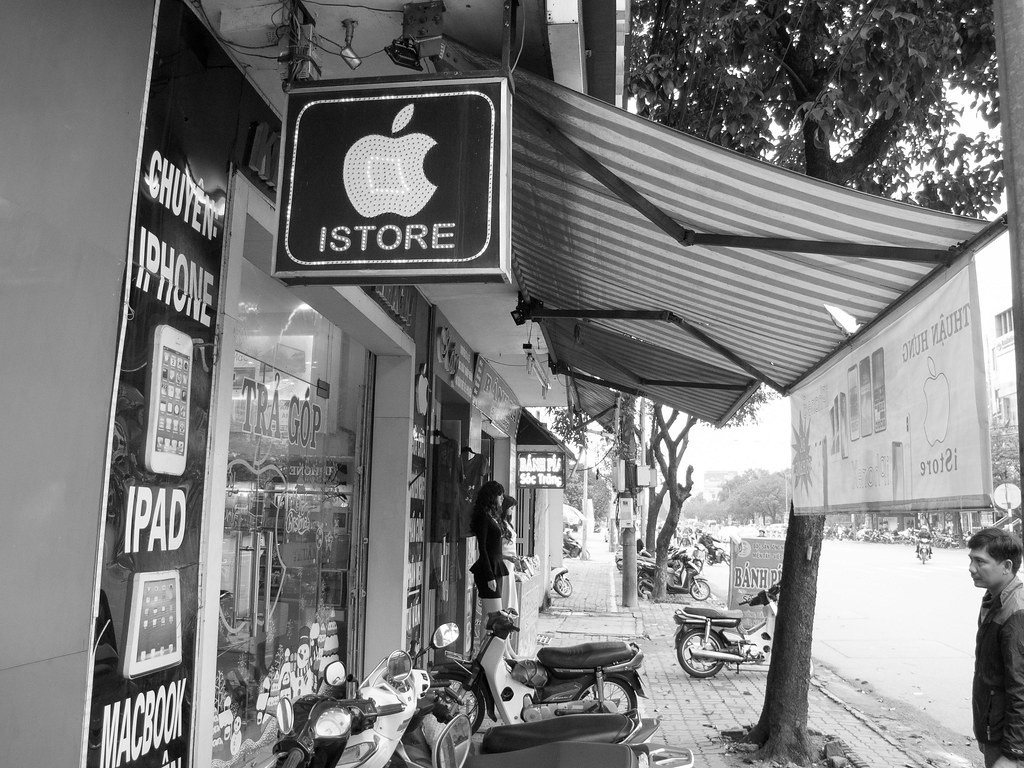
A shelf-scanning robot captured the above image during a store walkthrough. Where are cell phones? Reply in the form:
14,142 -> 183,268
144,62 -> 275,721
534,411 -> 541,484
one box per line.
127,570 -> 182,680
143,324 -> 193,476
829,347 -> 887,460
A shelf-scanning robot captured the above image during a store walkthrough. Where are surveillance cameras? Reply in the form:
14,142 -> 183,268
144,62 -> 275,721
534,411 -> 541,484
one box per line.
341,47 -> 362,70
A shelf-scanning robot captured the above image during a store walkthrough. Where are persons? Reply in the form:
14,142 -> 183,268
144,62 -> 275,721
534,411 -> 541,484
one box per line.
495,493 -> 520,662
757,530 -> 766,538
916,525 -> 933,560
656,523 -> 724,562
968,528 -> 1024,768
469,480 -> 510,645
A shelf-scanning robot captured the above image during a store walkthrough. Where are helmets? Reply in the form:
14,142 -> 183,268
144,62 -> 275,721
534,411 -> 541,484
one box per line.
702,527 -> 712,537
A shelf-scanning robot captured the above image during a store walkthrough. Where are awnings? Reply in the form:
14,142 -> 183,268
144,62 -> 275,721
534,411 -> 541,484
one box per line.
448,34 -> 1000,430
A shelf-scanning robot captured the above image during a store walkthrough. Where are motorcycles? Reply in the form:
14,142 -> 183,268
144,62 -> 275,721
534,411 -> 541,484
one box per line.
432,607 -> 648,718
916,536 -> 934,565
323,621 -> 660,768
563,541 -> 590,560
670,575 -> 782,679
253,648 -> 694,768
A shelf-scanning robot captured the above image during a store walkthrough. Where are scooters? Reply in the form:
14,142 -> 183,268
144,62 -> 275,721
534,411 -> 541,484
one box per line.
549,564 -> 574,598
614,536 -> 730,601
